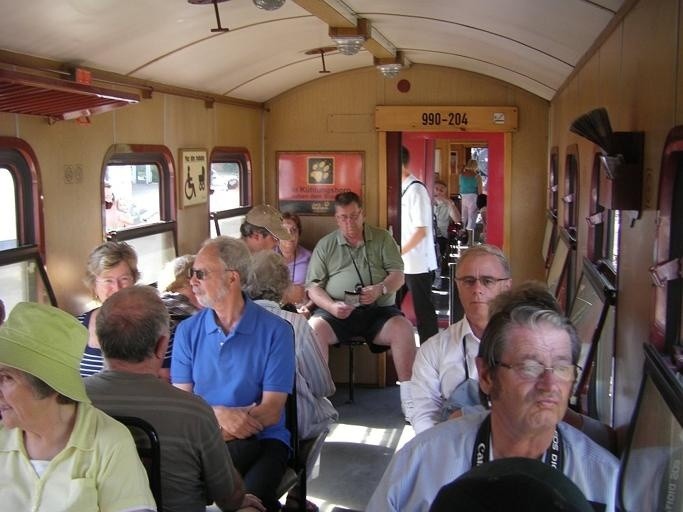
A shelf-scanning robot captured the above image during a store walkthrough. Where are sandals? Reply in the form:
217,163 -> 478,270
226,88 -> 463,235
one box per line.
282,497 -> 318,511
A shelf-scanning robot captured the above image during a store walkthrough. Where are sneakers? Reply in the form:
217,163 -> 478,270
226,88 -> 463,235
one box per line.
401,399 -> 416,426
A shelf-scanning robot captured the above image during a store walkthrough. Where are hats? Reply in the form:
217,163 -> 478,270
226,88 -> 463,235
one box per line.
246,205 -> 291,241
1,302 -> 92,403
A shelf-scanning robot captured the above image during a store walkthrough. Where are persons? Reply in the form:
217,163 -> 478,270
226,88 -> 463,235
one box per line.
433,181 -> 460,286
363,310 -> 623,510
400,144 -> 437,342
75,190 -> 417,512
410,244 -> 512,433
0,302 -> 156,511
447,282 -> 618,455
458,157 -> 481,232
169,235 -> 298,512
80,283 -> 267,510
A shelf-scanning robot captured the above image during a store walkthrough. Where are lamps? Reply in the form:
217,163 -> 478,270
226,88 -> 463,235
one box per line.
376,64 -> 402,78
253,0 -> 285,11
330,36 -> 366,56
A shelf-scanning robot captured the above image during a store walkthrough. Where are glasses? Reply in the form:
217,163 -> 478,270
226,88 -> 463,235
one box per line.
453,276 -> 507,289
495,358 -> 582,381
187,267 -> 238,279
